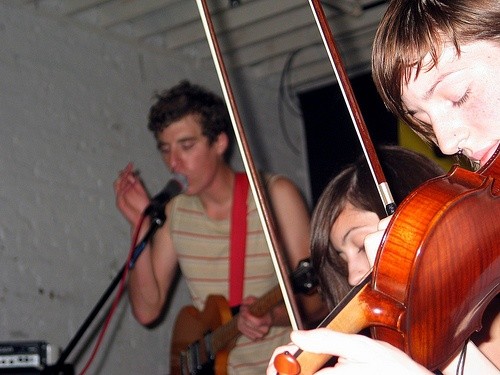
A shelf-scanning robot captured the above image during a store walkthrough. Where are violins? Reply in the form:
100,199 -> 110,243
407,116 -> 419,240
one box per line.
274,143 -> 500,375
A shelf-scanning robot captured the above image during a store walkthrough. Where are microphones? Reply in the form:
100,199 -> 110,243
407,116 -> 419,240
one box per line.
142,172 -> 188,216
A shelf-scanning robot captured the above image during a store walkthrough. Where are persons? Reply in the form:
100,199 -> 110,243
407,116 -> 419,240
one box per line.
266,0 -> 500,375
116,80 -> 335,375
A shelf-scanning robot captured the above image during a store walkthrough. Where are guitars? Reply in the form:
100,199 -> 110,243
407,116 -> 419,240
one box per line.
169,257 -> 319,375
